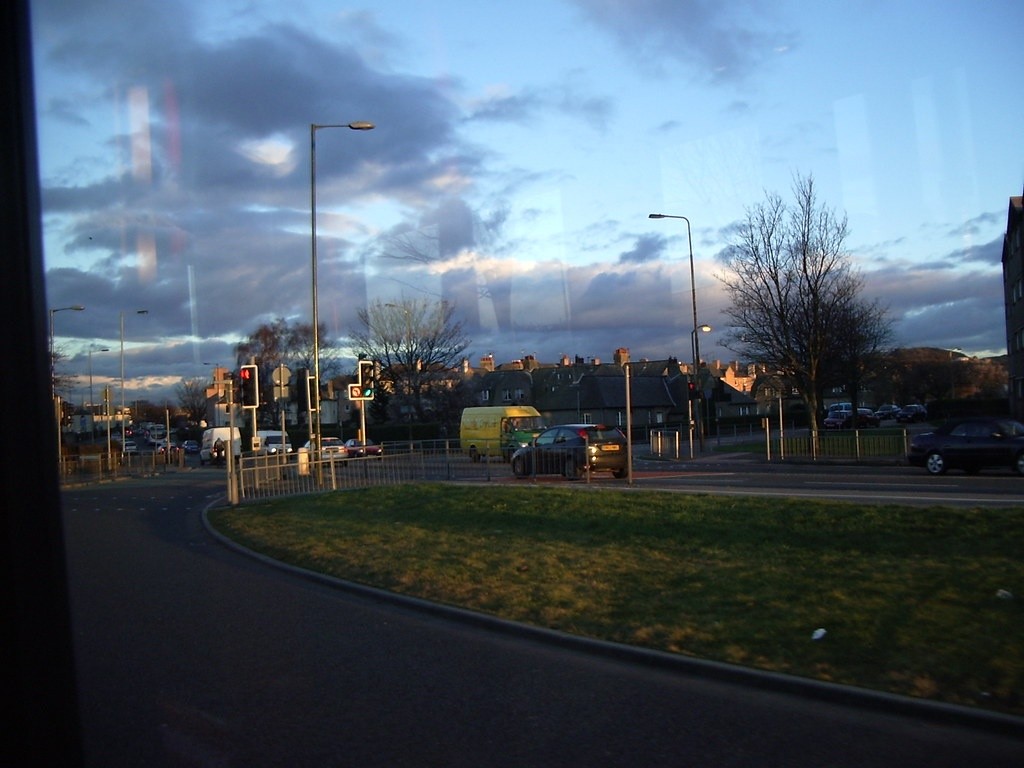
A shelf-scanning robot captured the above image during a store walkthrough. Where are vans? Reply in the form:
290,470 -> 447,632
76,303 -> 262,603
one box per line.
200,426 -> 242,466
458,405 -> 547,465
256,430 -> 293,460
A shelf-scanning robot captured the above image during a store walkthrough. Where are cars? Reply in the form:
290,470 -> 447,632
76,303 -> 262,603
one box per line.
306,436 -> 347,469
181,439 -> 201,454
510,423 -> 631,480
905,414 -> 1024,479
346,438 -> 382,458
823,403 -> 928,430
124,421 -> 180,456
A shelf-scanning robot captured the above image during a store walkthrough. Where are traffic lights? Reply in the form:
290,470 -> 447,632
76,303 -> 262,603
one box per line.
349,360 -> 377,400
239,364 -> 259,409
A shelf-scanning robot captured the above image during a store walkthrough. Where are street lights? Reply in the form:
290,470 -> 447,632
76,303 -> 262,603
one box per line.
88,348 -> 109,447
949,346 -> 963,400
648,213 -> 707,450
118,308 -> 150,457
690,324 -> 710,456
49,305 -> 86,400
202,361 -> 221,427
383,303 -> 415,456
310,120 -> 378,485
